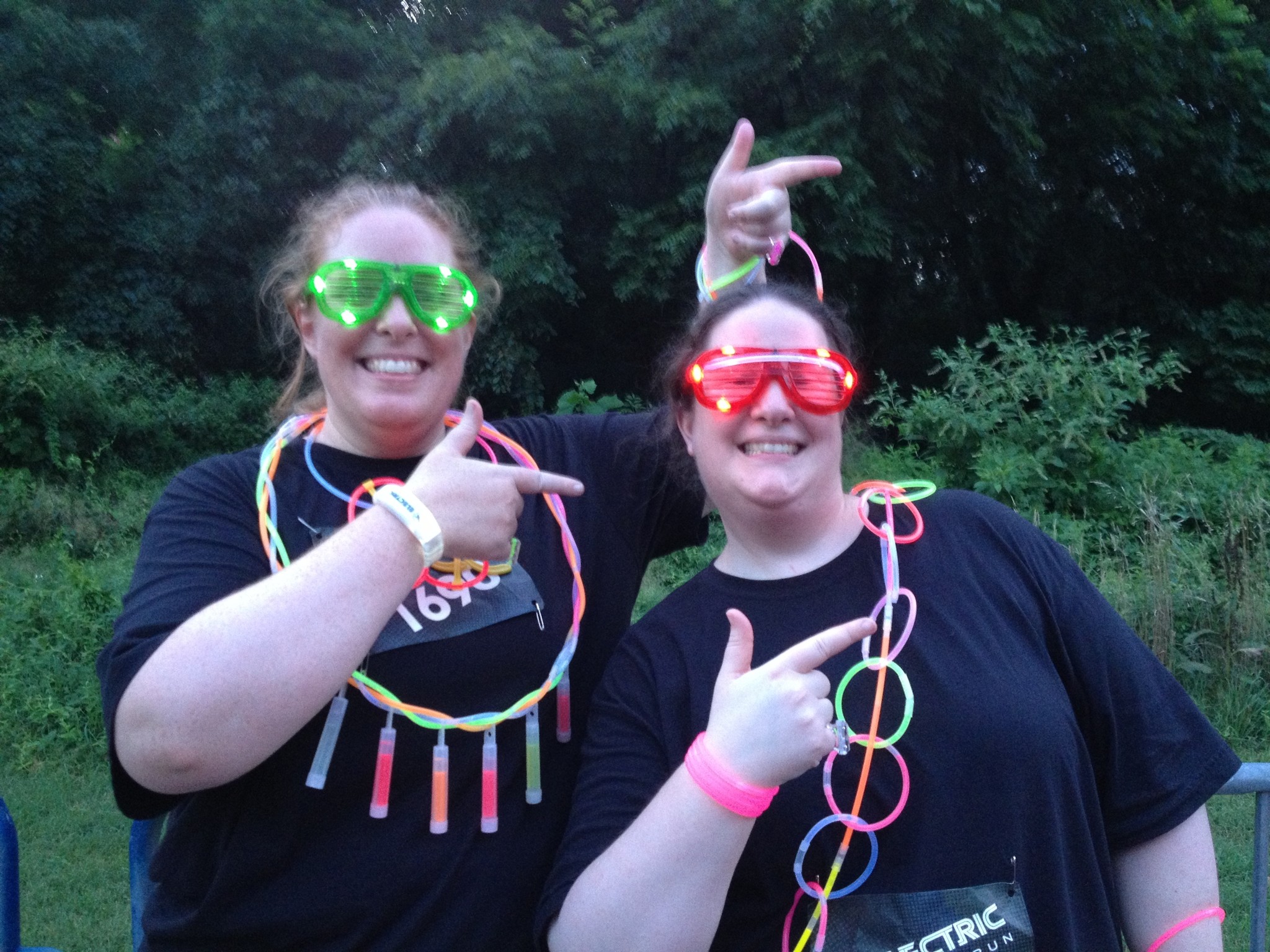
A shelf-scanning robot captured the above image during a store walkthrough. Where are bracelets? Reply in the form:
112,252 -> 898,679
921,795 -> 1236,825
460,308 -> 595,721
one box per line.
372,482 -> 444,568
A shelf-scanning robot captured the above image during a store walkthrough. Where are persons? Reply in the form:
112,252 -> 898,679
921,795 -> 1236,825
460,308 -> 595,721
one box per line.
96,118 -> 842,952
531,280 -> 1243,952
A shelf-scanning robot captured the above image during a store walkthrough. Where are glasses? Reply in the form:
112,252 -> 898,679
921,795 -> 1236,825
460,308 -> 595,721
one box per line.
685,345 -> 858,418
307,255 -> 478,335
